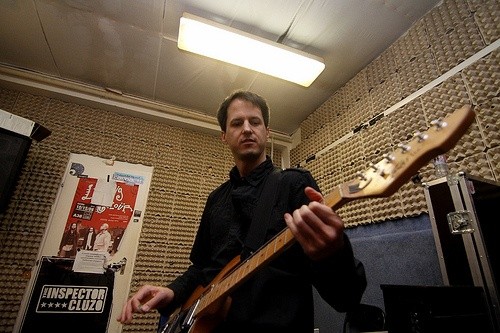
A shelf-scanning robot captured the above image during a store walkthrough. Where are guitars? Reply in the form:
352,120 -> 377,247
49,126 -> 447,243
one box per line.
156,104 -> 476,333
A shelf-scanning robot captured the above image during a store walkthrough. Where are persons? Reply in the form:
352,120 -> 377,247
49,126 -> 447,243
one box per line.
92,223 -> 110,254
112,228 -> 121,253
76,221 -> 81,240
61,222 -> 77,259
117,91 -> 368,333
83,227 -> 95,250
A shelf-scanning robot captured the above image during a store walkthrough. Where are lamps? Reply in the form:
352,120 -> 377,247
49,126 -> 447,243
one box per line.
177,11 -> 325,88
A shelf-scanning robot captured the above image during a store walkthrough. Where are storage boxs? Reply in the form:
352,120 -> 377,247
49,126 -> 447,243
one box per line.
422,172 -> 500,333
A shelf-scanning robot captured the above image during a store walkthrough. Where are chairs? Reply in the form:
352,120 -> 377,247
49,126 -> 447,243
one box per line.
342,304 -> 386,333
